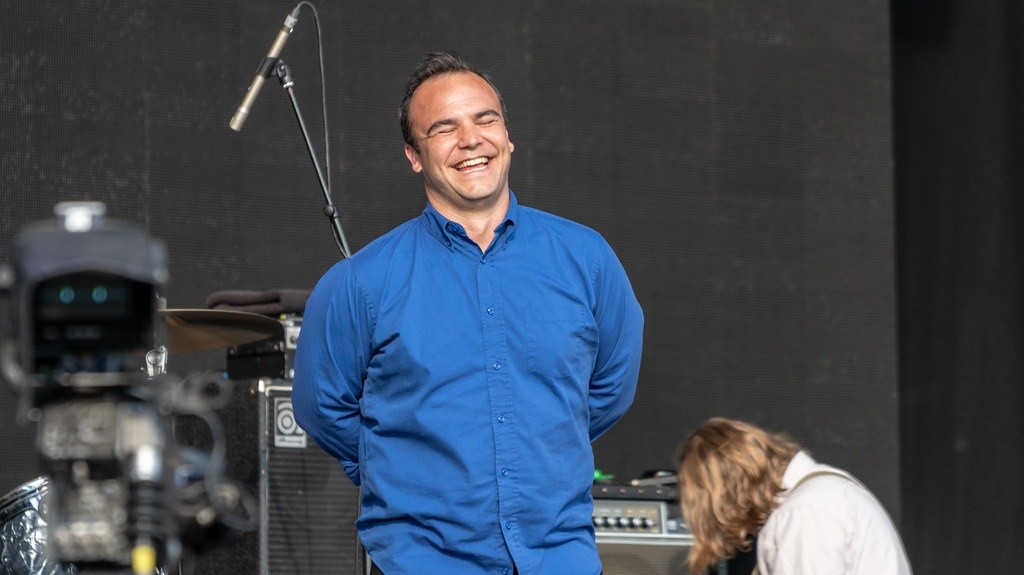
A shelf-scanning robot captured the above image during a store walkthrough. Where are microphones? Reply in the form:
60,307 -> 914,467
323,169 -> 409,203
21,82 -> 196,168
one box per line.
229,10 -> 298,132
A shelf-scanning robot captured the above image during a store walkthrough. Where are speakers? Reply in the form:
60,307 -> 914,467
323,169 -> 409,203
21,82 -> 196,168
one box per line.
594,533 -> 729,575
169,376 -> 363,575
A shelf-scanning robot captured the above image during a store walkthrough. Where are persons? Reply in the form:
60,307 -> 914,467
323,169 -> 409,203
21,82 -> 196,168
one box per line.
679,416 -> 913,575
291,49 -> 645,575
